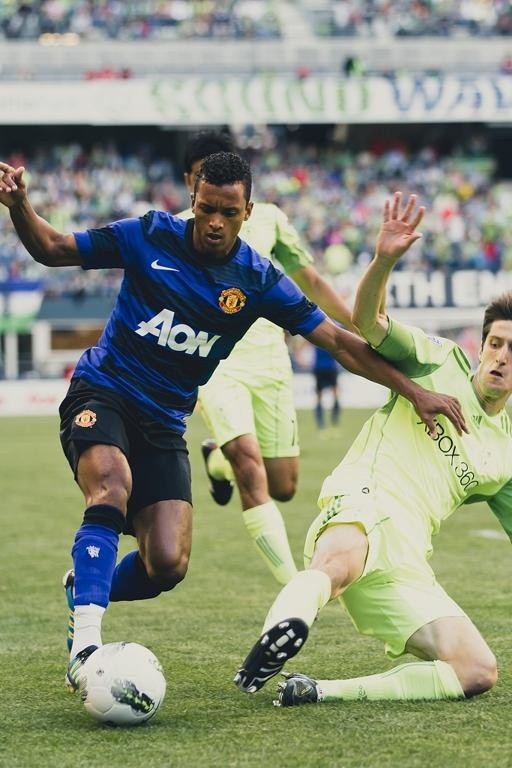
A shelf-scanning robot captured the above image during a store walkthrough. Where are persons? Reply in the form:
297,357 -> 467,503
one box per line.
232,188 -> 511,707
312,336 -> 343,431
0,153 -> 472,691
171,127 -> 368,590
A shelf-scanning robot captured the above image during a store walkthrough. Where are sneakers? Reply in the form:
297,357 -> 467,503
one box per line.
200,440 -> 234,507
62,569 -> 76,651
232,618 -> 309,697
274,672 -> 323,709
67,645 -> 101,691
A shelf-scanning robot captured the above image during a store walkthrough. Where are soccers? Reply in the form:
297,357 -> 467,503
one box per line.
78,641 -> 167,728
